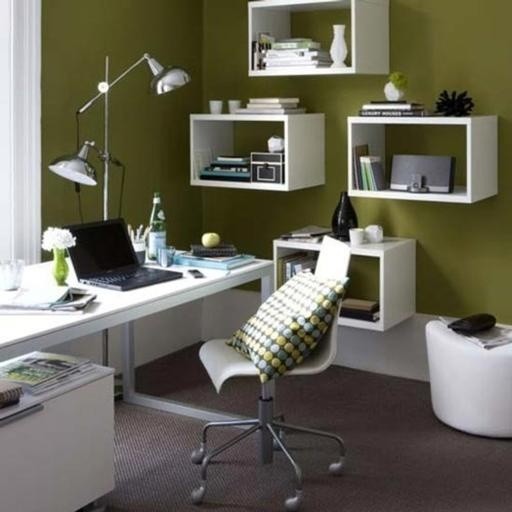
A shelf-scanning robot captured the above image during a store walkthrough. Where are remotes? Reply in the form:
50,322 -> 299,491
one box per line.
188,267 -> 206,280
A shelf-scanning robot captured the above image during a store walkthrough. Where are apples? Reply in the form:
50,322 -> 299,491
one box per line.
201,232 -> 221,248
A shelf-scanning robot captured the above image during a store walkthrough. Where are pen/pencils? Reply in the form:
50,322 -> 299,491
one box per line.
126,222 -> 152,241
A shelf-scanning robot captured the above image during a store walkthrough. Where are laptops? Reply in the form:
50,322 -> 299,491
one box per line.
60,216 -> 182,293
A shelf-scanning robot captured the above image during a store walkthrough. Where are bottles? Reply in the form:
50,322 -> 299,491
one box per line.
329,24 -> 348,68
150,191 -> 167,259
331,191 -> 358,240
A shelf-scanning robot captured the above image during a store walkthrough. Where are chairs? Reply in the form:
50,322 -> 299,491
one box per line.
190,235 -> 347,512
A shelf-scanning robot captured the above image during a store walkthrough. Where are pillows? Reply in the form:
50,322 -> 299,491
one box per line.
225,267 -> 360,383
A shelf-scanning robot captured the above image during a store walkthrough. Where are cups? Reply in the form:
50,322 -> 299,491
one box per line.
349,228 -> 364,245
0,258 -> 23,291
208,100 -> 241,114
132,242 -> 145,265
158,246 -> 175,266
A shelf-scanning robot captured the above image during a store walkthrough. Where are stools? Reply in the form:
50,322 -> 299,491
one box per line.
425,320 -> 512,438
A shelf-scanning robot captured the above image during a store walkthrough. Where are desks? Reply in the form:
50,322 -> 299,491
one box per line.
0,246 -> 275,437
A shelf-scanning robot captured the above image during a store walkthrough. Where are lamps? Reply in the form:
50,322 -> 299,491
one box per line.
46,51 -> 190,222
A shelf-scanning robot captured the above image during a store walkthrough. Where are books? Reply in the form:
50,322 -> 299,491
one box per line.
199,157 -> 251,182
0,287 -> 97,315
353,103 -> 425,192
238,96 -> 307,115
275,225 -> 333,290
0,350 -> 101,396
173,252 -> 255,270
260,37 -> 332,69
340,298 -> 380,322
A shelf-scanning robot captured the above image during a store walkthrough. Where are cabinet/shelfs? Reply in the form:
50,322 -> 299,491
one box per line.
273,231 -> 418,331
247,0 -> 389,77
192,114 -> 325,192
0,353 -> 115,512
346,114 -> 498,200
